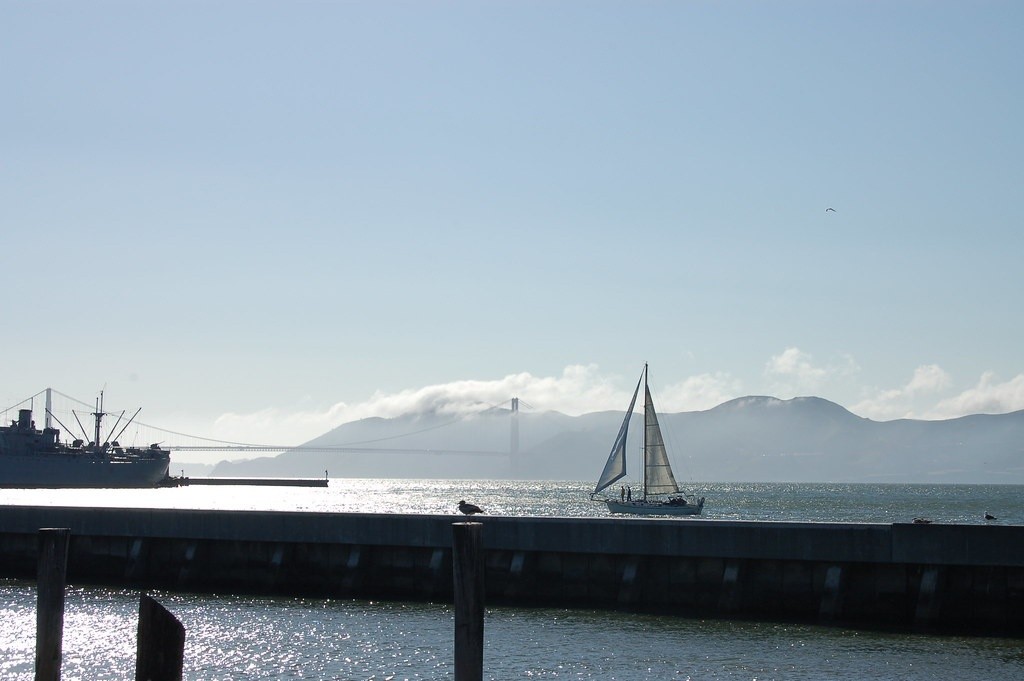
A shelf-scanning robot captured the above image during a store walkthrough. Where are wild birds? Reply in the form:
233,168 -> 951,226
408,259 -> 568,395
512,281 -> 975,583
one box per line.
911,517 -> 933,525
457,500 -> 484,523
984,511 -> 998,525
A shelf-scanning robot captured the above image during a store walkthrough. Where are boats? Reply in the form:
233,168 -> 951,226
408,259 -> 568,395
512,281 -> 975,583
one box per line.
0,389 -> 190,489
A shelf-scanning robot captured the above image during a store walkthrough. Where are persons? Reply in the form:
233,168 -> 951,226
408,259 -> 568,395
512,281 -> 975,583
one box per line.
626,486 -> 632,501
621,486 -> 625,502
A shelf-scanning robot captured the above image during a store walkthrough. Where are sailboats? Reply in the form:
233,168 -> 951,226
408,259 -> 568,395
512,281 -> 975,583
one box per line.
588,360 -> 706,515
